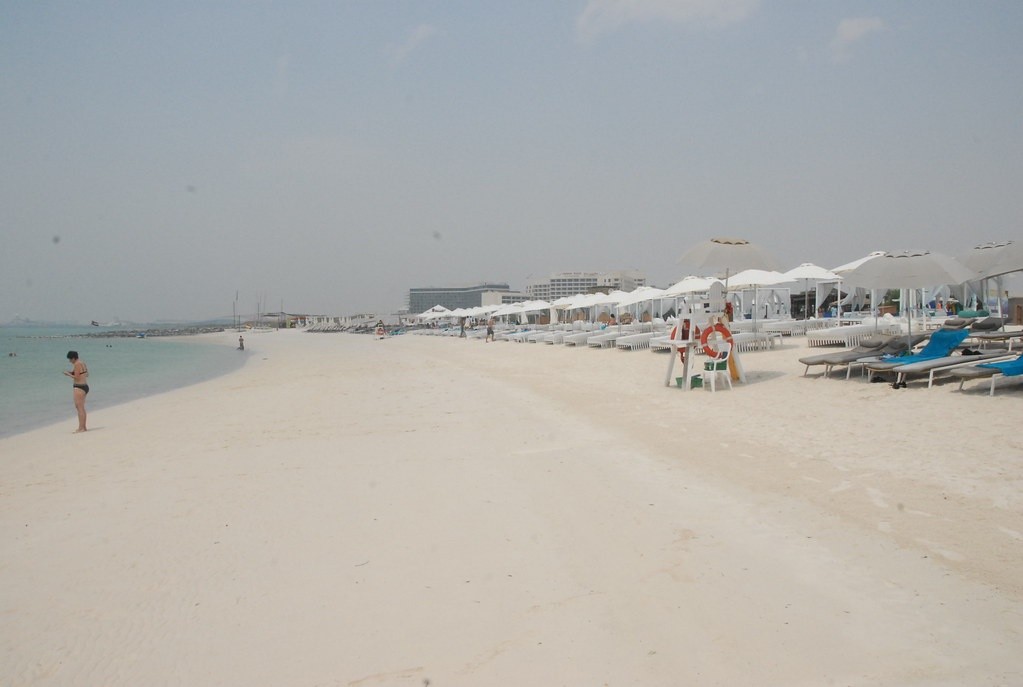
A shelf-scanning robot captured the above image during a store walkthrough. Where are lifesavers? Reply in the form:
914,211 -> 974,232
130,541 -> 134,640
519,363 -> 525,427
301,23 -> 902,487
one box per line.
669,320 -> 700,353
700,326 -> 735,358
378,328 -> 384,335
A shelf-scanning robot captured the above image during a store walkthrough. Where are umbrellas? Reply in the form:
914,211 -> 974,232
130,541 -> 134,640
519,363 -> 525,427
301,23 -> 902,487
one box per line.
955,240 -> 1023,319
417,290 -> 628,325
831,251 -> 975,356
616,262 -> 843,332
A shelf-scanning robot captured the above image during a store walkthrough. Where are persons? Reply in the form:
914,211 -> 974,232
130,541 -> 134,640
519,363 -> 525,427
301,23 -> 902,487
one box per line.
63,351 -> 89,434
239,336 -> 244,346
485,317 -> 496,342
376,320 -> 385,340
831,306 -> 837,317
516,321 -> 520,325
607,313 -> 618,326
426,321 -> 440,329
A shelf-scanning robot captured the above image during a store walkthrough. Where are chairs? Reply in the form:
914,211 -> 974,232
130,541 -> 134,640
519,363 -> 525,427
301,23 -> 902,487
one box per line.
301,316 -> 1023,397
